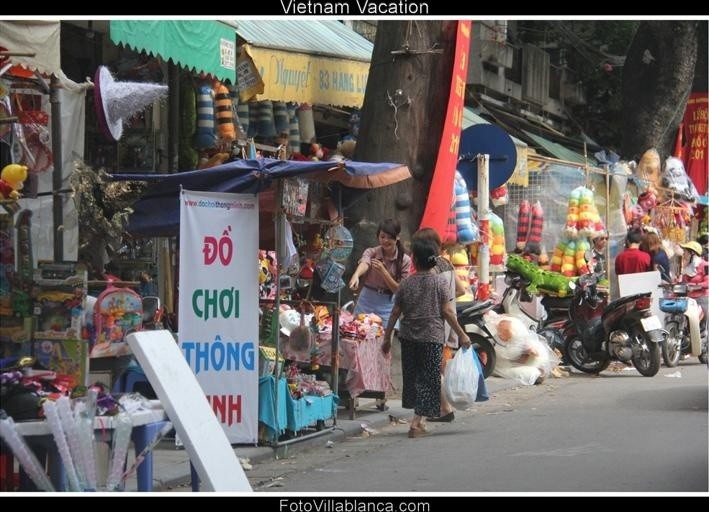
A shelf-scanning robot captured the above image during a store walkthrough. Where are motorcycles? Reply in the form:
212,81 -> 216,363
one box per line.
499,250 -> 708,376
343,287 -> 503,379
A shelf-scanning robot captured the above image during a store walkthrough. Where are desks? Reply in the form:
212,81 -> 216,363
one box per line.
280,332 -> 392,419
0,399 -> 199,492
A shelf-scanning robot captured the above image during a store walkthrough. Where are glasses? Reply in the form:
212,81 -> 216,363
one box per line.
601,236 -> 609,240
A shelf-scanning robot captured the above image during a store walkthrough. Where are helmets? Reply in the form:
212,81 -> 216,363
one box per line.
678,241 -> 702,256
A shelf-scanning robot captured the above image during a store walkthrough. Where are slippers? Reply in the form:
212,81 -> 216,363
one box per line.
409,423 -> 434,438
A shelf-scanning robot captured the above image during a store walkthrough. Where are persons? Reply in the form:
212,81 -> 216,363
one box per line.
349,217 -> 412,412
593,227 -> 610,280
614,226 -> 709,369
410,227 -> 468,423
380,237 -> 473,438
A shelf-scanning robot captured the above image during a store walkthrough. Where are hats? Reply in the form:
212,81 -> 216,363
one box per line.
73,166 -> 147,240
93,64 -> 169,143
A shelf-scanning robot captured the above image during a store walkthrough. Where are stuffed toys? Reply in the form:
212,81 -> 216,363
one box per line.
199,151 -> 231,169
0,209 -> 148,422
0,164 -> 30,201
290,139 -> 358,161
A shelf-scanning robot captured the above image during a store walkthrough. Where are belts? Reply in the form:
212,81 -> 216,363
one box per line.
364,282 -> 393,295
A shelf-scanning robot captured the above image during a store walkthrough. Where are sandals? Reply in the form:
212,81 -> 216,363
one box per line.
426,411 -> 455,422
